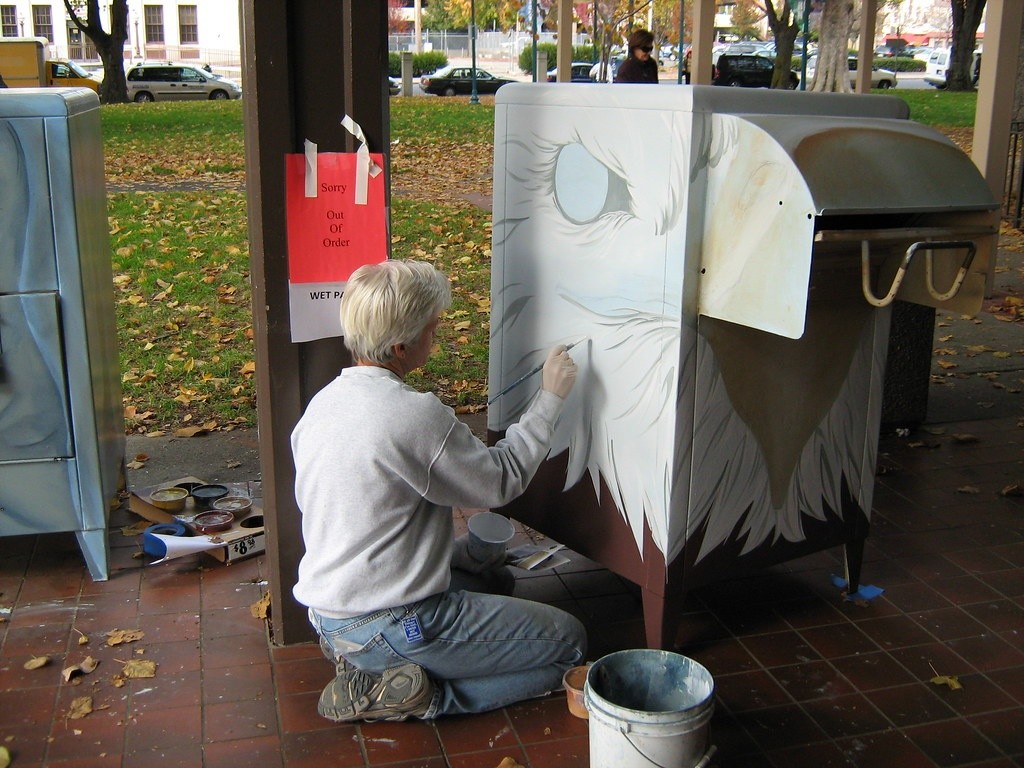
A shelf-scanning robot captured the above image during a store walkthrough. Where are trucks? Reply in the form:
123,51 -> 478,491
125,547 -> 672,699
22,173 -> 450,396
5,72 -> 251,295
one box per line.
0,37 -> 103,98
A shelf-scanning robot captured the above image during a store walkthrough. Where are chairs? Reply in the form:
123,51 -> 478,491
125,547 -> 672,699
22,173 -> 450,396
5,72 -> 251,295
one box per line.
467,72 -> 472,78
461,72 -> 465,78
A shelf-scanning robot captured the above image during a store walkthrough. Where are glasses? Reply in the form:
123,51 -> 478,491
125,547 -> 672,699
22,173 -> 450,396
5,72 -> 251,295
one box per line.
639,46 -> 654,52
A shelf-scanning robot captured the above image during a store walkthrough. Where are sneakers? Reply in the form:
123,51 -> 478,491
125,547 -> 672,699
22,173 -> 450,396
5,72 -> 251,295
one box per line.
317,633 -> 433,723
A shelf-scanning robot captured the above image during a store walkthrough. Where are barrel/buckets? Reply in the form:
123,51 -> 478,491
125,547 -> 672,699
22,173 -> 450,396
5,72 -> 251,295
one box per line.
584,649 -> 717,768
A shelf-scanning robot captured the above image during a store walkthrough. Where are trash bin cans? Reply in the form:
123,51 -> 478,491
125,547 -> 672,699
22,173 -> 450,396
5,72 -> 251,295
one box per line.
583,649 -> 718,768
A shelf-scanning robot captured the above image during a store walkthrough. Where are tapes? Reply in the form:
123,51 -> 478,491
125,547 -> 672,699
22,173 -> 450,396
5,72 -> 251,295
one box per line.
143,522 -> 187,558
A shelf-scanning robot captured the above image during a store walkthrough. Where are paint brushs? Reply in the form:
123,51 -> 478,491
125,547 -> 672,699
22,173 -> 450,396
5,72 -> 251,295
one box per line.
486,337 -> 587,407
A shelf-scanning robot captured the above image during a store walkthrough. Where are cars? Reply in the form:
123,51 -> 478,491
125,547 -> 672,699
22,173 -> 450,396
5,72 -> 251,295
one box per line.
924,45 -> 983,90
801,54 -> 898,91
386,76 -> 401,96
620,35 -> 817,79
546,62 -> 596,83
869,43 -> 919,60
419,64 -> 521,97
123,64 -> 242,101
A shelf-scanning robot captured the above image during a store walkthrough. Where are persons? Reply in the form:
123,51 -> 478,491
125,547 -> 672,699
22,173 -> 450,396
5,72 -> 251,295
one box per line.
719,36 -> 724,42
204,65 -> 211,72
972,54 -> 982,84
290,259 -> 589,722
681,50 -> 692,85
615,29 -> 658,83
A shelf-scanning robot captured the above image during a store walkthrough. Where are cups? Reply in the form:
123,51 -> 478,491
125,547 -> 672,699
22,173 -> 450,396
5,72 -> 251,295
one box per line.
562,667 -> 589,719
466,511 -> 515,562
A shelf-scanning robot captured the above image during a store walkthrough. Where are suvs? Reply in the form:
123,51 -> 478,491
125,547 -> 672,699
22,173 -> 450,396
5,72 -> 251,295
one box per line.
716,53 -> 799,90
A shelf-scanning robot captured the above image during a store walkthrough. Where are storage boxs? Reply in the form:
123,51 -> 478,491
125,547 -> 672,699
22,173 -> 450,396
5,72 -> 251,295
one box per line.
128,477 -> 265,564
